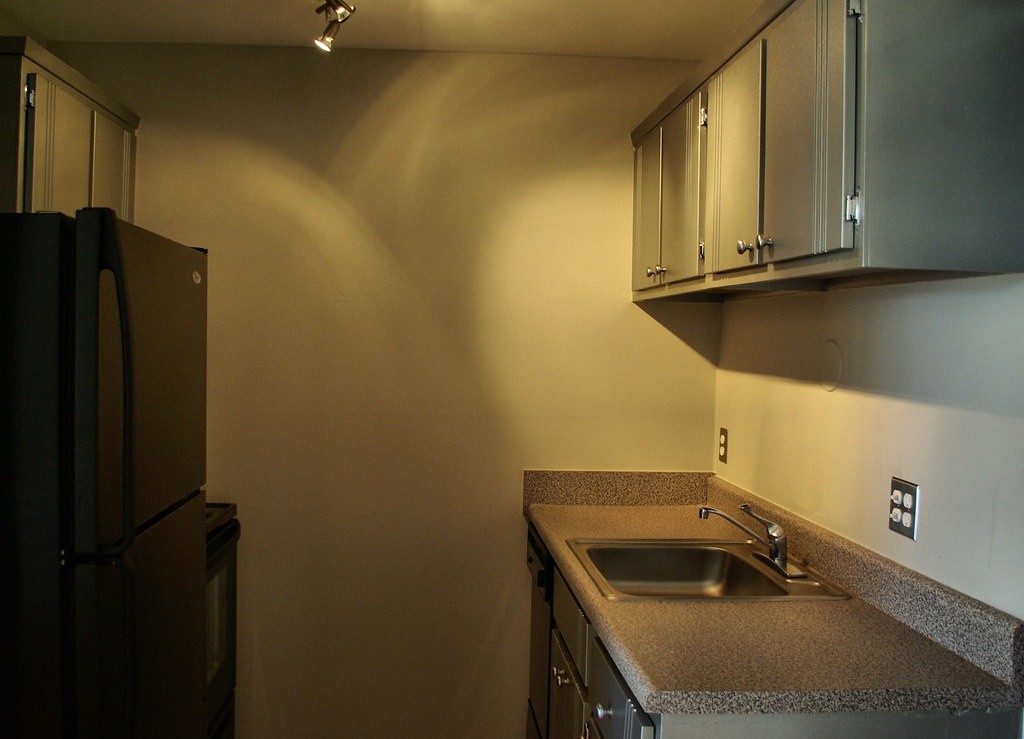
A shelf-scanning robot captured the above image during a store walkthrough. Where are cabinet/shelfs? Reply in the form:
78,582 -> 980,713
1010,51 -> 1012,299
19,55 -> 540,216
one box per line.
704,0 -> 1024,294
0,33 -> 142,225
548,562 -> 1023,738
629,91 -> 797,305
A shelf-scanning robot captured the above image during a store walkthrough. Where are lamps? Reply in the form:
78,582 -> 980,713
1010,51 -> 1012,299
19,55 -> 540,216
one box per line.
312,1 -> 356,51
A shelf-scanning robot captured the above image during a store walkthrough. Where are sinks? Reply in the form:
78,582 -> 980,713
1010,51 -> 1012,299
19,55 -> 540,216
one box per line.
565,535 -> 791,599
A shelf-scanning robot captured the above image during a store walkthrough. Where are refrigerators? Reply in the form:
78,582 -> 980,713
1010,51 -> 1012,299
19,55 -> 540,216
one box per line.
0,205 -> 209,739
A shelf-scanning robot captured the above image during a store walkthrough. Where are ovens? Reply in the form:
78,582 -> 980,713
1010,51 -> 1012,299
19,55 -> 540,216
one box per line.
205,517 -> 241,739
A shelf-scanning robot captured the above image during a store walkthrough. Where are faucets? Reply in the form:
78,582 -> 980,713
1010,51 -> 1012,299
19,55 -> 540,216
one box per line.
699,505 -> 787,557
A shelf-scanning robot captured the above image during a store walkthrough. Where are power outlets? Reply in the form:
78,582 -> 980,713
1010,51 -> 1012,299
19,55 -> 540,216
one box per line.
889,477 -> 920,542
719,428 -> 728,465
